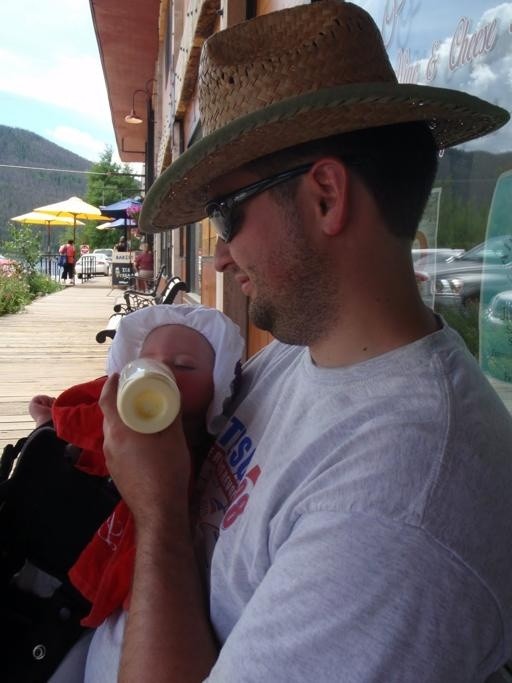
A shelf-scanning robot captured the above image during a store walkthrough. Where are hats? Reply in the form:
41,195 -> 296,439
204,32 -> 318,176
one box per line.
141,0 -> 508,232
106,303 -> 245,438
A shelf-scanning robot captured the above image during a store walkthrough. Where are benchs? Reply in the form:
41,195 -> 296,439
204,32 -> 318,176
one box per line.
95,262 -> 187,345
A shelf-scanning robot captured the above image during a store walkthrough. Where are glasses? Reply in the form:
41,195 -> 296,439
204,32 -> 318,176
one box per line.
202,162 -> 310,240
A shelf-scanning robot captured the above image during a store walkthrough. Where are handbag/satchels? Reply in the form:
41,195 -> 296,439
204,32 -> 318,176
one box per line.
57,254 -> 67,266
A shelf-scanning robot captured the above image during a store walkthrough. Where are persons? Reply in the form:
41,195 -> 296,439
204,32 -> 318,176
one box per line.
25,304 -> 247,429
59,239 -> 76,285
45,2 -> 512,683
114,235 -> 126,252
132,243 -> 153,293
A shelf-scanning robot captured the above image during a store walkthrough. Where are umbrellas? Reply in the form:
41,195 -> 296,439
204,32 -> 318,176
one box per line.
33,196 -> 113,244
101,197 -> 143,242
10,212 -> 86,276
108,217 -> 137,228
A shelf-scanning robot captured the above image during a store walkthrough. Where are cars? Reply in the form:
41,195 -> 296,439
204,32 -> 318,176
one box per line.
75,254 -> 110,279
413,235 -> 512,328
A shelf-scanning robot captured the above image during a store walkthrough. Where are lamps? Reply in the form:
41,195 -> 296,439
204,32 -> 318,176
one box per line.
126,87 -> 152,125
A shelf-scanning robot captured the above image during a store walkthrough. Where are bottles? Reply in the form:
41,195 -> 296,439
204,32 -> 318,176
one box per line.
116,357 -> 182,436
